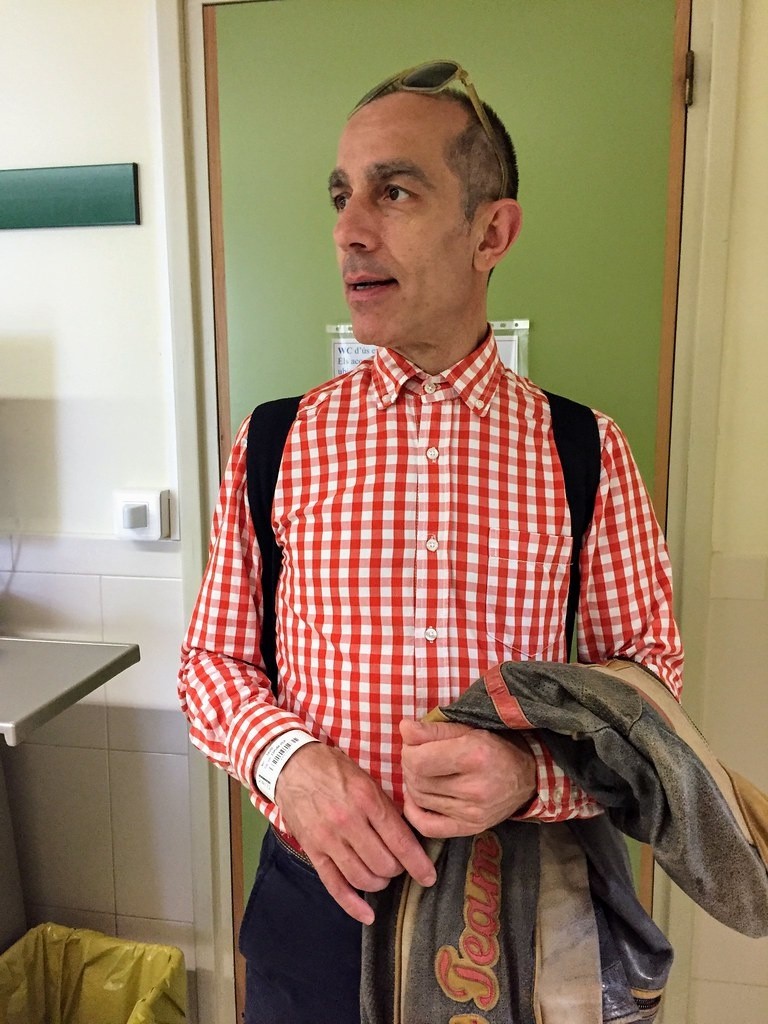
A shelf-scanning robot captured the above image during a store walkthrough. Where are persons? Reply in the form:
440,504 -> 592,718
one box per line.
176,59 -> 686,1024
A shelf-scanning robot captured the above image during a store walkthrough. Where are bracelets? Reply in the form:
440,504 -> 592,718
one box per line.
256,730 -> 322,806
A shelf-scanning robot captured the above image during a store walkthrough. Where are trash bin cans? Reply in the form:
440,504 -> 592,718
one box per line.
1,921 -> 193,1024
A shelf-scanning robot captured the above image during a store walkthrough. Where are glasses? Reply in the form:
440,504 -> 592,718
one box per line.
347,59 -> 508,200
